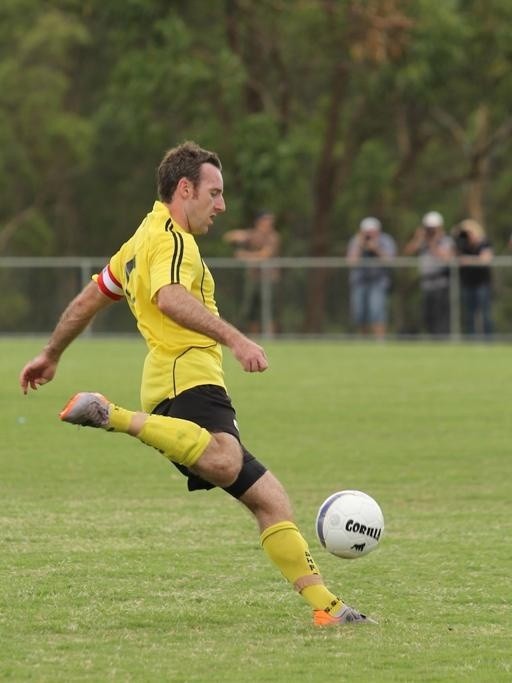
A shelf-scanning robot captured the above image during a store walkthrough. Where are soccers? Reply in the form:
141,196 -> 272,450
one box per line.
315,489 -> 384,558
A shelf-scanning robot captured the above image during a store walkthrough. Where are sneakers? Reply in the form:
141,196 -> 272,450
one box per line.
59,392 -> 112,428
313,606 -> 377,626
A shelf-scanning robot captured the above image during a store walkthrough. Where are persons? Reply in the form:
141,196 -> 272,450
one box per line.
345,211 -> 494,340
18,140 -> 384,629
222,212 -> 283,341
